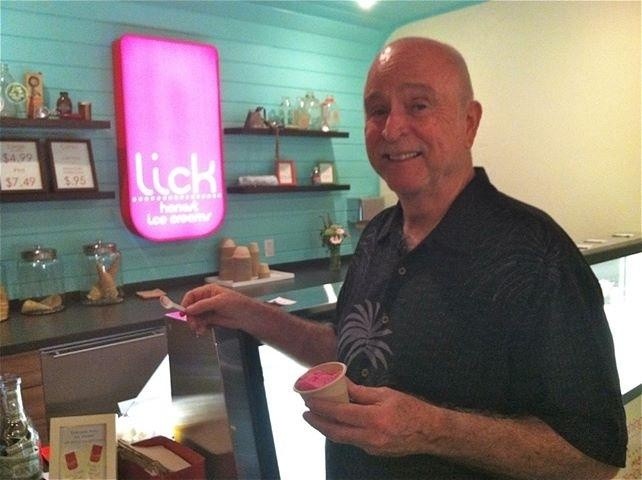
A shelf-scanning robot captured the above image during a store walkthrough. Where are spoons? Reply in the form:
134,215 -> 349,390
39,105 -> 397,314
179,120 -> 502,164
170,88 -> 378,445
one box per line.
158,296 -> 185,313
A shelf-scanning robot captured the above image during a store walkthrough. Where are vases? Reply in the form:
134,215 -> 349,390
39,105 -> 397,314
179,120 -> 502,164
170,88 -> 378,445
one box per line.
329,245 -> 341,271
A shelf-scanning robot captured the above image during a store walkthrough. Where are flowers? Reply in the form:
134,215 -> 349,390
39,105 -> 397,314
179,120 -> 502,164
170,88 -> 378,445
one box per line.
319,213 -> 351,253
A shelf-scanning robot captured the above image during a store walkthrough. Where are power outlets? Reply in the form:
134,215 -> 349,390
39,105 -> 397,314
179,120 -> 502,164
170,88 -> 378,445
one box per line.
264,240 -> 274,257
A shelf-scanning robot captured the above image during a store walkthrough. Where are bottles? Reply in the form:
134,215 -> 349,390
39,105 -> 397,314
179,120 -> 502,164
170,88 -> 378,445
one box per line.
0,62 -> 94,121
1,238 -> 126,321
244,91 -> 340,132
0,372 -> 44,479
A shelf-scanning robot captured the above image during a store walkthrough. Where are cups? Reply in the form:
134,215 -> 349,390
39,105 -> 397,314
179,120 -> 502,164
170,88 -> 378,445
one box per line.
292,361 -> 352,418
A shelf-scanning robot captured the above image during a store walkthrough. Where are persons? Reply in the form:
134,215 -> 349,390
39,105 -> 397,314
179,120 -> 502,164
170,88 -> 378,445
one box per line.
178,32 -> 628,480
